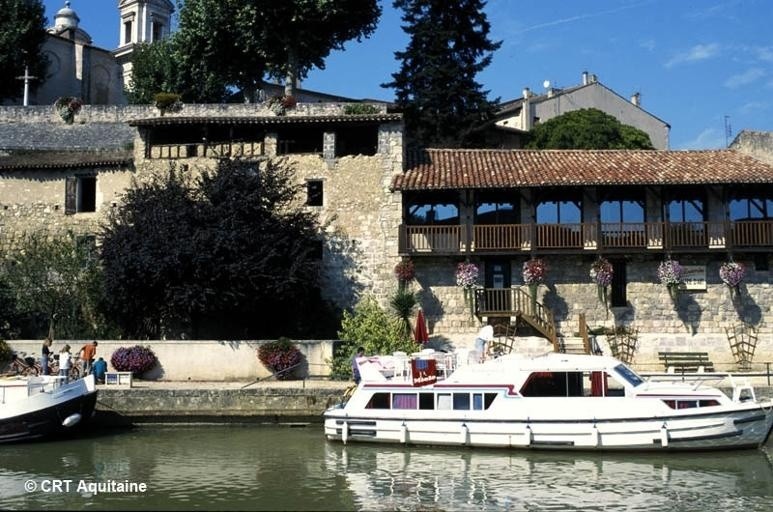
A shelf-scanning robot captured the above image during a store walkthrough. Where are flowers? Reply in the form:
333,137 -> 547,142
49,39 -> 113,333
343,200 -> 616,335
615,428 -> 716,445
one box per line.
258,340 -> 298,381
589,256 -> 614,287
719,259 -> 747,287
455,262 -> 480,288
522,259 -> 550,284
110,346 -> 156,375
394,258 -> 415,281
656,259 -> 684,284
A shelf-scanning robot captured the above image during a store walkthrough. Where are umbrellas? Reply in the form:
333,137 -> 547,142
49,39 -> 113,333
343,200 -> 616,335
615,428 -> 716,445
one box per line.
413,309 -> 428,350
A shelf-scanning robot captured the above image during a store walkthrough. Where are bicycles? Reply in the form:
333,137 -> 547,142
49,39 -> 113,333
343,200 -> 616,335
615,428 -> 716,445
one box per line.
4,351 -> 81,380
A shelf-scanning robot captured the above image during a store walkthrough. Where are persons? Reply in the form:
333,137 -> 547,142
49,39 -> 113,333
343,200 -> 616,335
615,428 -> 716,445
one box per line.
78,340 -> 97,378
92,357 -> 107,385
474,324 -> 492,359
40,338 -> 52,376
57,344 -> 70,387
351,347 -> 368,385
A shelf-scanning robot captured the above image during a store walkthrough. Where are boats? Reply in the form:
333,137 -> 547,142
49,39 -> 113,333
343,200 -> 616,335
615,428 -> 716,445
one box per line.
0,372 -> 98,445
321,324 -> 773,448
